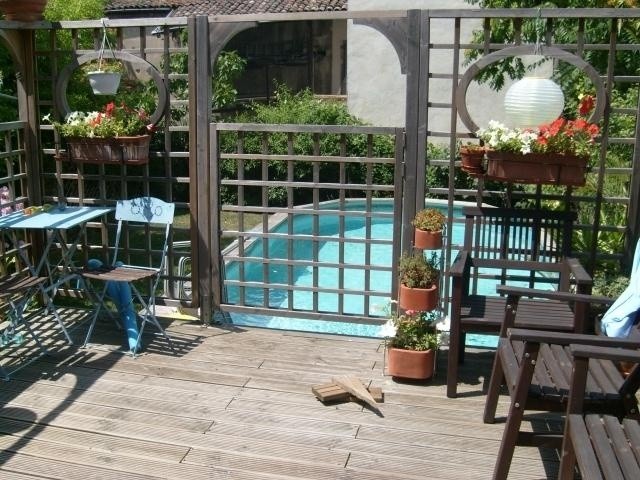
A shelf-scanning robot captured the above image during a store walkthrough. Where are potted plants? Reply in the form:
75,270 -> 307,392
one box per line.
413,207 -> 445,250
460,140 -> 484,174
400,252 -> 440,311
78,19 -> 121,95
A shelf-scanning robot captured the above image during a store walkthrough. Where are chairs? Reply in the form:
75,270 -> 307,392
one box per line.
559,344 -> 639,480
483,285 -> 640,480
447,207 -> 595,399
1,235 -> 50,383
78,198 -> 178,357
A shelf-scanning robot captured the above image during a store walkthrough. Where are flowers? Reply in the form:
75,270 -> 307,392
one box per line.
42,103 -> 158,136
390,306 -> 442,353
474,97 -> 600,156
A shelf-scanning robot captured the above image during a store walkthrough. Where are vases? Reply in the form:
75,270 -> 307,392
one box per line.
387,344 -> 436,380
486,151 -> 588,185
67,137 -> 153,164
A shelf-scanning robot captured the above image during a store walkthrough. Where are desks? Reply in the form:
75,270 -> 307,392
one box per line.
0,204 -> 120,350
